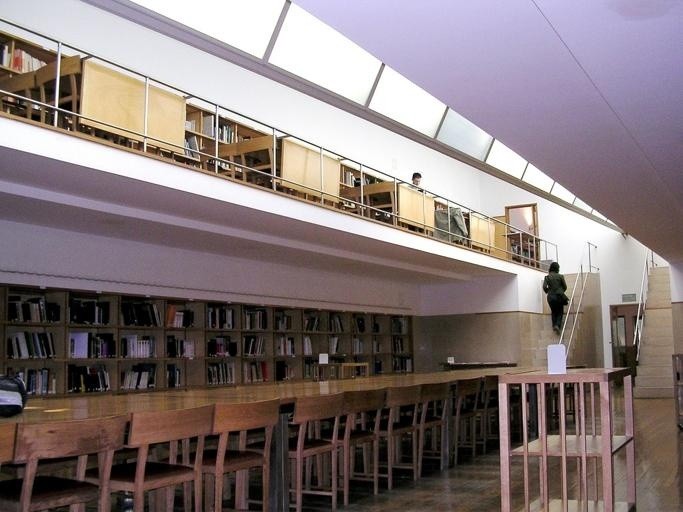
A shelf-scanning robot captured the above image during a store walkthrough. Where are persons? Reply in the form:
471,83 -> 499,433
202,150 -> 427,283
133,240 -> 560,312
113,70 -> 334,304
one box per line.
543,262 -> 567,335
408,172 -> 424,233
354,178 -> 370,218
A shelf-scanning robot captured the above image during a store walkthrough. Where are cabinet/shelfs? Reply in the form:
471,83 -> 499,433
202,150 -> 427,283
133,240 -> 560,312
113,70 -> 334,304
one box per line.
498,366 -> 636,511
0,282 -> 412,401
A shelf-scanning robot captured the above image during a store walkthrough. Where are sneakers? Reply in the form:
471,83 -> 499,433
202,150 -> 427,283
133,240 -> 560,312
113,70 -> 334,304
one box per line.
552,325 -> 560,335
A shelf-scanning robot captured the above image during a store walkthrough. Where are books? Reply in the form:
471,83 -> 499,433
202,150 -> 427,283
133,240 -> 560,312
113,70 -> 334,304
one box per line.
329,315 -> 345,377
165,304 -> 194,388
244,309 -> 269,382
121,301 -> 161,389
345,171 -> 355,187
372,318 -> 383,375
68,299 -> 115,393
208,307 -> 237,384
275,314 -> 295,381
352,316 -> 366,376
305,317 -> 322,377
8,296 -> 61,394
2,44 -> 47,74
391,318 -> 404,371
185,115 -> 244,172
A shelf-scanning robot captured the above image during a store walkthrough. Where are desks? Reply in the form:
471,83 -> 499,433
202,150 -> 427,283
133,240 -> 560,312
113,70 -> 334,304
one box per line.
79,60 -> 496,256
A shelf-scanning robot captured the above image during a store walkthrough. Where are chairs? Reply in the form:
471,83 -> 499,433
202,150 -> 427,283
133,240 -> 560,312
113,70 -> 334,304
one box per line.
369,384 -> 421,490
319,386 -> 387,507
243,393 -> 344,511
79,403 -> 213,511
418,373 -> 580,479
1,55 -> 112,141
1,413 -> 129,511
162,400 -> 279,511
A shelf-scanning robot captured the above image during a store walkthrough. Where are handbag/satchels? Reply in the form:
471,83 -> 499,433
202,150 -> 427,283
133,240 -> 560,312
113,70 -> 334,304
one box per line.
561,293 -> 568,305
